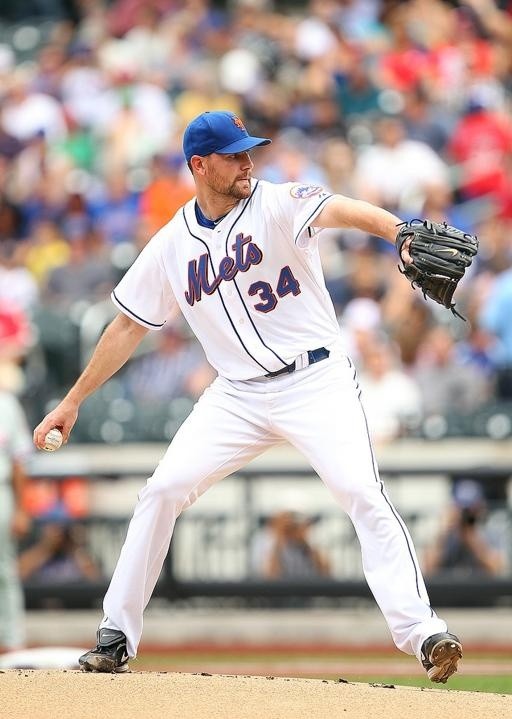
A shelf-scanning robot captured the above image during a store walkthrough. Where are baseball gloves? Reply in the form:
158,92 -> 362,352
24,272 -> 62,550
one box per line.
396,222 -> 479,311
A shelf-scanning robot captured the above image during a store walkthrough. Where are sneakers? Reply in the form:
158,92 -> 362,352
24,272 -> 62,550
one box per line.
420,634 -> 462,684
79,629 -> 130,673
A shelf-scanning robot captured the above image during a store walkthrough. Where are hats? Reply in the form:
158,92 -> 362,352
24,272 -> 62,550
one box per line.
183,110 -> 271,163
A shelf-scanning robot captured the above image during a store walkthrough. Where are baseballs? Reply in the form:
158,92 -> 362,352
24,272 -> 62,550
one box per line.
43,428 -> 65,453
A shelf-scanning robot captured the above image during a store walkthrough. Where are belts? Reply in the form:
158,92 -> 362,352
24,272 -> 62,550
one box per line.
264,346 -> 329,378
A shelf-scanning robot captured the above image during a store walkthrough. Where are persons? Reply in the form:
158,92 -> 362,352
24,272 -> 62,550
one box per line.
31,112 -> 478,682
1,1 -> 512,614
